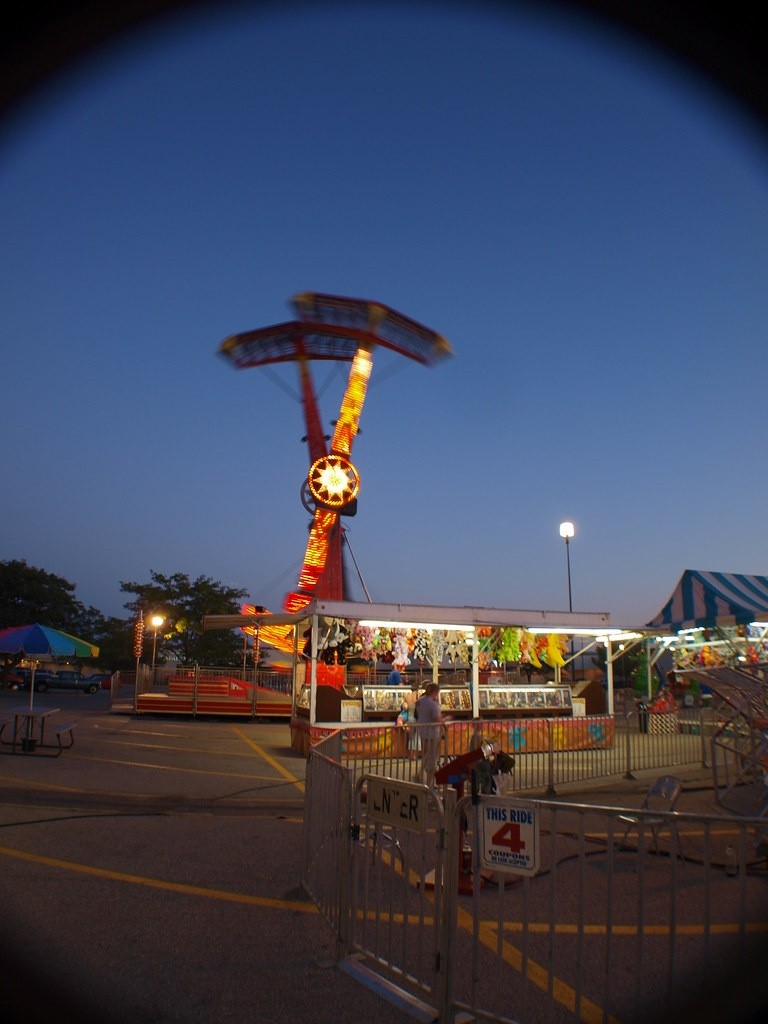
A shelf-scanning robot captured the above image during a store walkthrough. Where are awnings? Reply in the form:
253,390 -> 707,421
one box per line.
200,614 -> 312,661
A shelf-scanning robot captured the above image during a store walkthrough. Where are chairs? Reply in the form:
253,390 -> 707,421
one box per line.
616,775 -> 688,867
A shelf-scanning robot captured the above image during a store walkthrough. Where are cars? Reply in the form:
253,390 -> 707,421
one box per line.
0,664 -> 34,692
88,674 -> 122,690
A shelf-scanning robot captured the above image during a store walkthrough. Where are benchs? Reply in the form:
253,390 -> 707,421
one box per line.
50,717 -> 80,757
0,715 -> 16,734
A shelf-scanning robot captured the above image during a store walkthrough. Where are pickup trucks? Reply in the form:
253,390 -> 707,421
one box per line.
34,670 -> 100,695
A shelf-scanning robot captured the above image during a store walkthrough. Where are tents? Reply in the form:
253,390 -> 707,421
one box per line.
643,570 -> 768,628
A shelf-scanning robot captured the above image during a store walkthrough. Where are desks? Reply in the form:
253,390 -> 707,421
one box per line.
0,705 -> 61,755
289,711 -> 618,763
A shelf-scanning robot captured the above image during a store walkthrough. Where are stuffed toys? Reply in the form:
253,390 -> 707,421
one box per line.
302,625 -> 571,670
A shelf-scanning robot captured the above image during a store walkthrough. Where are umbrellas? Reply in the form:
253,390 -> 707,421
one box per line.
0,623 -> 100,737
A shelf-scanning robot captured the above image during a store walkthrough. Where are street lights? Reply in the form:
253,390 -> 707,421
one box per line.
559,522 -> 575,613
149,615 -> 165,686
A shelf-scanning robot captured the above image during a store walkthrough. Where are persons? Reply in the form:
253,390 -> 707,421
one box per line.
396,680 -> 451,789
469,717 -> 485,794
388,661 -> 408,685
